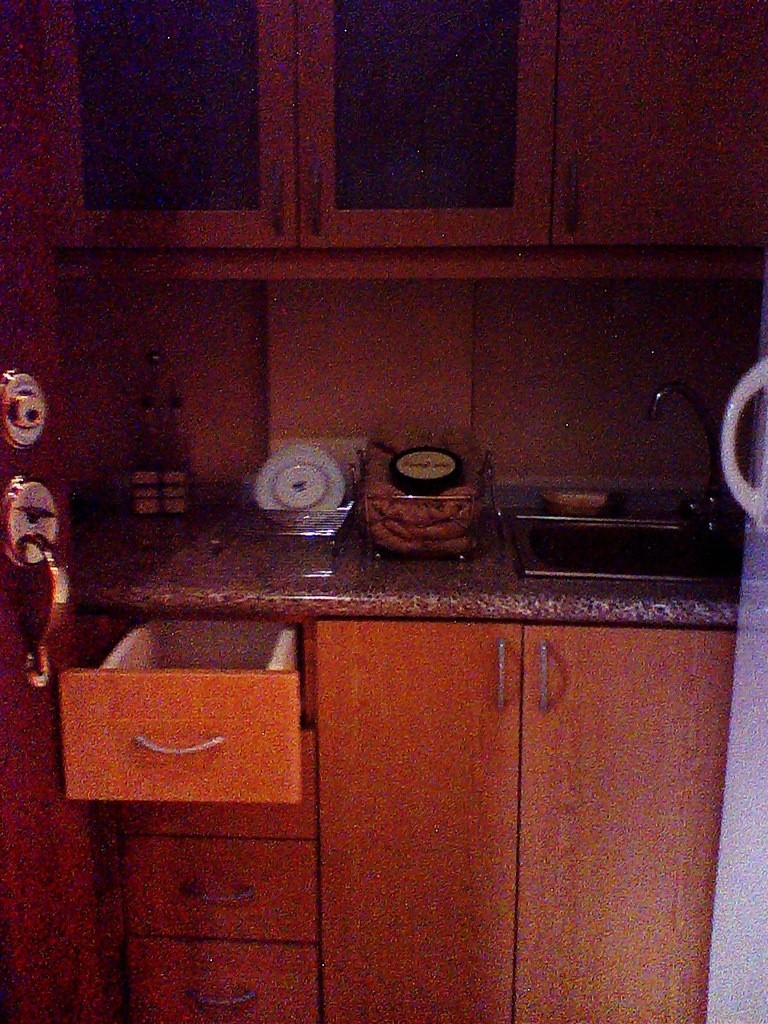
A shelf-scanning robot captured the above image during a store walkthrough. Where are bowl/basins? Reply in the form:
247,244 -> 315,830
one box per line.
539,486 -> 616,517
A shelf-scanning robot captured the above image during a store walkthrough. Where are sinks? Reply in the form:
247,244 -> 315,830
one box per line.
504,514 -> 741,582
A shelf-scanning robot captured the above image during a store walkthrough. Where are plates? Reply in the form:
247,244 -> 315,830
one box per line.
254,445 -> 344,523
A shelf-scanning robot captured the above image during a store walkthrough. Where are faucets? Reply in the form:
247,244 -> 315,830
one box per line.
648,382 -> 722,496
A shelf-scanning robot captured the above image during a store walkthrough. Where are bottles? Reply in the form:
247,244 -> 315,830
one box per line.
138,349 -> 170,405
161,399 -> 190,515
132,398 -> 161,513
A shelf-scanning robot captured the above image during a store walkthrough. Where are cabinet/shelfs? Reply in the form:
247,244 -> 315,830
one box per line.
312,613 -> 739,1023
32,0 -> 556,286
58,613 -> 324,1024
548,0 -> 768,282
